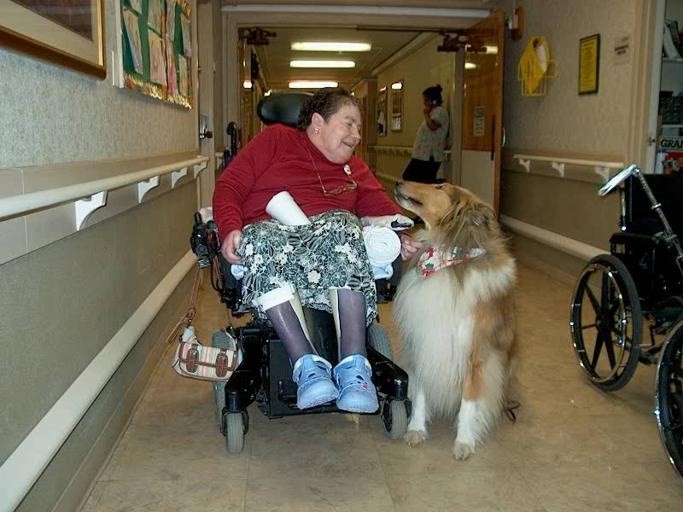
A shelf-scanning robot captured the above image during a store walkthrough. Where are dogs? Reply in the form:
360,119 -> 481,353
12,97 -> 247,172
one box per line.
392,179 -> 517,461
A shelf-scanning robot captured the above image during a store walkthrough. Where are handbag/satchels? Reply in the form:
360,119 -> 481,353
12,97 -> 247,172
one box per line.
163,300 -> 244,382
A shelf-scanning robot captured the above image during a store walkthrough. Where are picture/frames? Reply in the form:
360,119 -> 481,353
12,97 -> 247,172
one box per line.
0,0 -> 107,82
578,35 -> 599,96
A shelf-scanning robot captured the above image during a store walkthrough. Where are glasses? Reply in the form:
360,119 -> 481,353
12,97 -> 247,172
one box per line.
317,174 -> 358,196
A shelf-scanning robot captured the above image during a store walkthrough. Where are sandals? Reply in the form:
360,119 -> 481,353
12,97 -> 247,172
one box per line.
335,353 -> 379,414
292,354 -> 339,409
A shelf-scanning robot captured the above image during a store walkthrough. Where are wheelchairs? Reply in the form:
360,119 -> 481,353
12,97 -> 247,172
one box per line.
182,86 -> 413,452
564,164 -> 683,476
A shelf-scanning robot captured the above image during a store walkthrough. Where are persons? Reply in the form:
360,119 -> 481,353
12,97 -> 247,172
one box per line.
213,86 -> 423,416
402,84 -> 450,185
